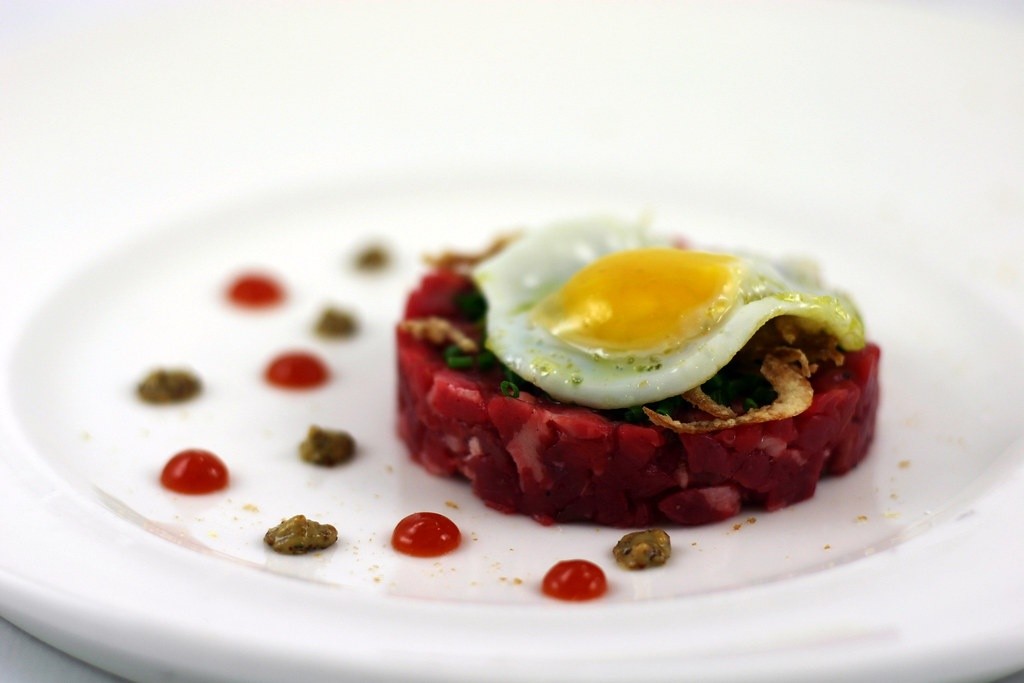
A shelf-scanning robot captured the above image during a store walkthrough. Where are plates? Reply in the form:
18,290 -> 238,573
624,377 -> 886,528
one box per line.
0,0 -> 1024,683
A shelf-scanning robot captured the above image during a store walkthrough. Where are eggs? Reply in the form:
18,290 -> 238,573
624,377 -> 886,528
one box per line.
467,209 -> 866,408
